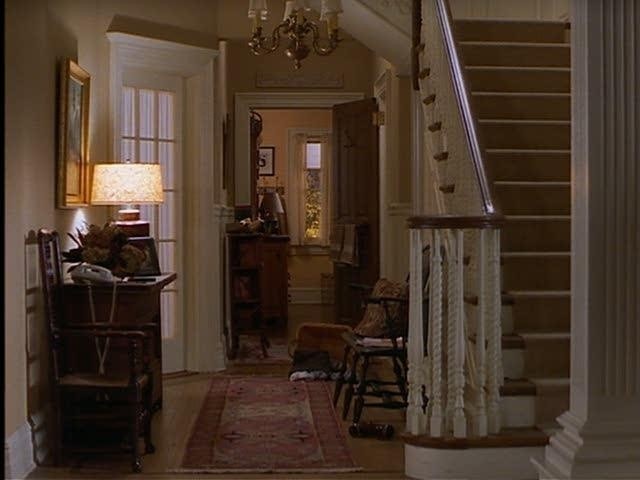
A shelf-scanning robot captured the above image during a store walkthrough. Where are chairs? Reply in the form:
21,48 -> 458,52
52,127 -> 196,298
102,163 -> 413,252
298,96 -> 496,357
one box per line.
334,243 -> 445,426
38,228 -> 157,473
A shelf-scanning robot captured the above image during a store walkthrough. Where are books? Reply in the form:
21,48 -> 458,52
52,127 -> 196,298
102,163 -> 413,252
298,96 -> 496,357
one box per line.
361,336 -> 407,350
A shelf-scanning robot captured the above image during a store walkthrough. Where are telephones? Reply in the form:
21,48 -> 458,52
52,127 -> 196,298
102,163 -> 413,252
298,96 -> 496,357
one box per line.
71,263 -> 111,283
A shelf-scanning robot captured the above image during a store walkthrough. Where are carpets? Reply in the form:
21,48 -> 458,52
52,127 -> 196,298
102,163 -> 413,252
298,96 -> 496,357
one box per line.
229,333 -> 295,365
179,374 -> 363,474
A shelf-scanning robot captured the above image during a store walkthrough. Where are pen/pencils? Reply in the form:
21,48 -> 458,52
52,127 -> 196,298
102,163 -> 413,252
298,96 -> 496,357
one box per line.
130,278 -> 157,281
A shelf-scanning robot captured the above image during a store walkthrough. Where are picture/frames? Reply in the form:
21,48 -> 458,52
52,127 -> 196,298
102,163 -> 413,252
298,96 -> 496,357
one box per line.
258,147 -> 274,176
54,58 -> 91,210
123,237 -> 162,277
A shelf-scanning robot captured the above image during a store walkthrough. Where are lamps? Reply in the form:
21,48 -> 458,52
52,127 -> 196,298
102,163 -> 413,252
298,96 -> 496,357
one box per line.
259,192 -> 284,234
89,160 -> 166,237
247,0 -> 344,69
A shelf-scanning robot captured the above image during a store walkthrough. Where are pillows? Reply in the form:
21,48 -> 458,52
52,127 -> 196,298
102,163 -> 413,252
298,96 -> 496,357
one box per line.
354,278 -> 409,337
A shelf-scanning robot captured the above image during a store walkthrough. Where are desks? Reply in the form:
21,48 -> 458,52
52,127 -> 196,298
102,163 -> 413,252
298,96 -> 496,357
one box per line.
227,234 -> 291,360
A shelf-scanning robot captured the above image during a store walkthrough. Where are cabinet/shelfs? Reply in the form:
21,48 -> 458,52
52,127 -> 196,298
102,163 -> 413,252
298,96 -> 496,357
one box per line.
55,271 -> 176,414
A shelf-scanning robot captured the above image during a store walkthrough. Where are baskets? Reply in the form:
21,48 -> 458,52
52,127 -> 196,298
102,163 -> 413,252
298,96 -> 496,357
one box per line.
286,322 -> 362,373
